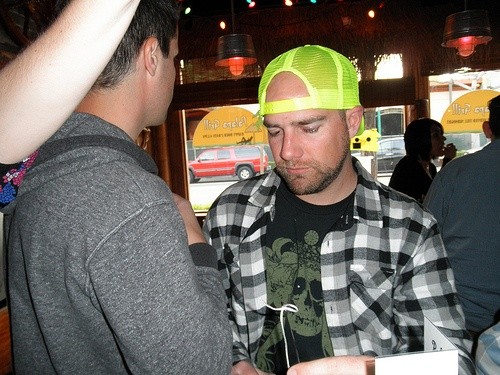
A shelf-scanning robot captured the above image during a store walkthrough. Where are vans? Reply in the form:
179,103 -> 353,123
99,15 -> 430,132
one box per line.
187,146 -> 268,183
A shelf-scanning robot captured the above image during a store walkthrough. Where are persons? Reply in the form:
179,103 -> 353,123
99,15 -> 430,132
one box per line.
0,0 -> 500,375
203,44 -> 476,375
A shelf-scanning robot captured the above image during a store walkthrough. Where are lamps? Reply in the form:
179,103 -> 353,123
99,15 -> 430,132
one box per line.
440,0 -> 494,57
215,0 -> 257,75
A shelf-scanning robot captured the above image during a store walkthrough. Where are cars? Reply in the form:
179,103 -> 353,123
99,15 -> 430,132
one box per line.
350,137 -> 440,173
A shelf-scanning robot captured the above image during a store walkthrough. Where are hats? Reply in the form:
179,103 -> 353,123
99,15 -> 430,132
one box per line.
256,44 -> 365,136
